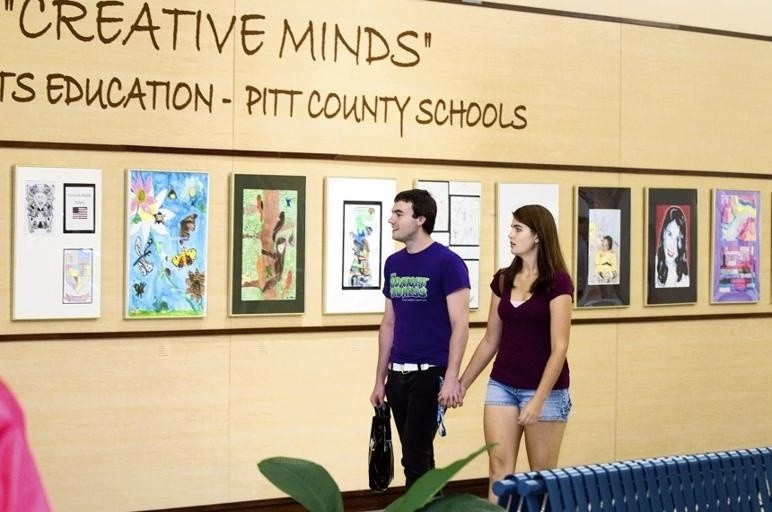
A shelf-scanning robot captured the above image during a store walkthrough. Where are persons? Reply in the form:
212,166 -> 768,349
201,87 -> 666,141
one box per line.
370,189 -> 473,511
442,203 -> 575,510
592,236 -> 620,284
654,205 -> 690,290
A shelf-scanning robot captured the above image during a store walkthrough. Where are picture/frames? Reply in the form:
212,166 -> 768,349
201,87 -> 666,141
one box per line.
228,174 -> 306,317
573,187 -> 632,310
642,186 -> 698,306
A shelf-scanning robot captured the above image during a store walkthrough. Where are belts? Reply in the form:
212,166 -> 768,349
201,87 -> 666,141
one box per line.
388,360 -> 432,374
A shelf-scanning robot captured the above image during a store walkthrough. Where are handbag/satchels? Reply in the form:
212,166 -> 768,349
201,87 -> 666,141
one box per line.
366,401 -> 394,493
